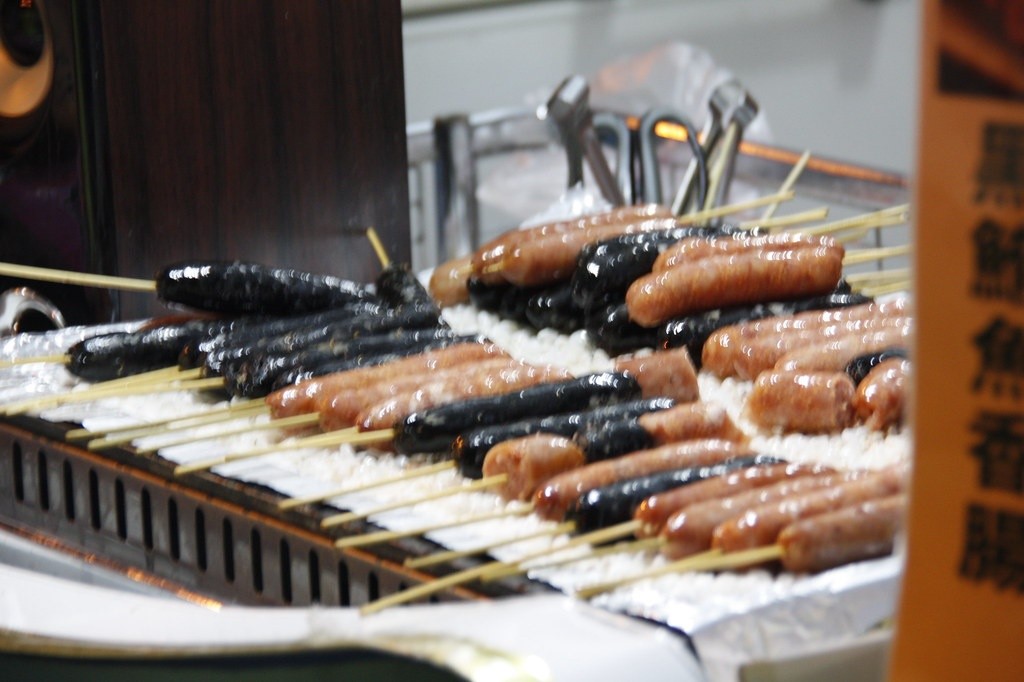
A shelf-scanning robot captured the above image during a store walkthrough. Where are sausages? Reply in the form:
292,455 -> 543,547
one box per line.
66,201 -> 916,574
